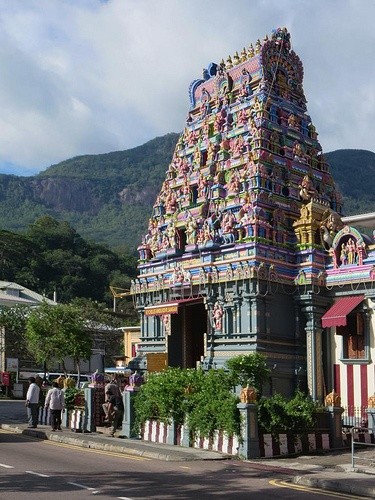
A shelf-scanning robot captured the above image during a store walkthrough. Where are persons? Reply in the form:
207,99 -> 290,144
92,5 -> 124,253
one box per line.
212,305 -> 223,330
24,377 -> 40,426
129,36 -> 364,292
44,383 -> 65,431
103,391 -> 123,438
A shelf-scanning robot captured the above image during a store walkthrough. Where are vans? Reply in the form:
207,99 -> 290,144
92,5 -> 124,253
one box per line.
43,373 -> 91,392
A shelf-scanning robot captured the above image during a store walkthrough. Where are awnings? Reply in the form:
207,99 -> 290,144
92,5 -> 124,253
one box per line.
144,297 -> 204,317
321,296 -> 364,328
